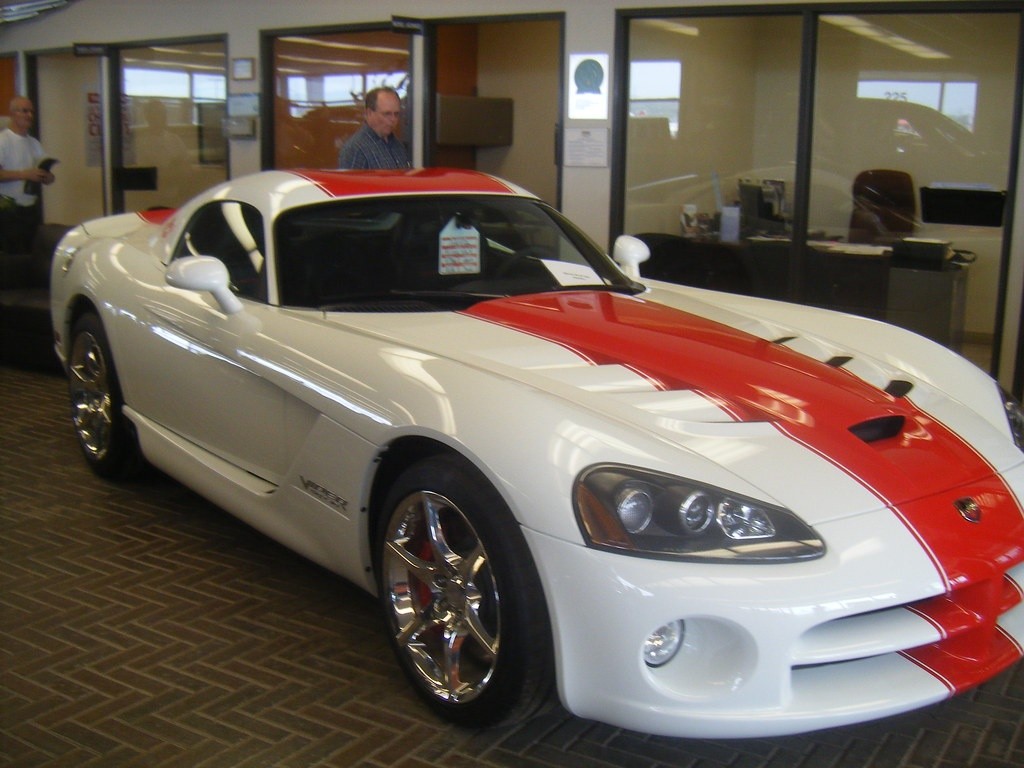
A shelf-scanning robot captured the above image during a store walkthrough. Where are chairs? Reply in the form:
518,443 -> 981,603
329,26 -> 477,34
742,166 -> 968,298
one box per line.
286,226 -> 491,304
847,169 -> 916,242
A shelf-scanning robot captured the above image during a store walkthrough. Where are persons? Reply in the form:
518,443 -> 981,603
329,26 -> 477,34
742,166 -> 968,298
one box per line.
1,96 -> 60,334
338,86 -> 414,170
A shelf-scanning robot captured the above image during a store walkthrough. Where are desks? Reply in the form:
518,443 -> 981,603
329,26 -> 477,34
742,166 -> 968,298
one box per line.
747,234 -> 894,256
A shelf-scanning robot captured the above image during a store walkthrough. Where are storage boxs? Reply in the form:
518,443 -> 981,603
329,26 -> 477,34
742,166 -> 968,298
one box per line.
889,267 -> 968,351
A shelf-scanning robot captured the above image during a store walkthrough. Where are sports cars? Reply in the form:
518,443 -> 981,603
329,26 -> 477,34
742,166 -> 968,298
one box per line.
48,168 -> 1024,745
